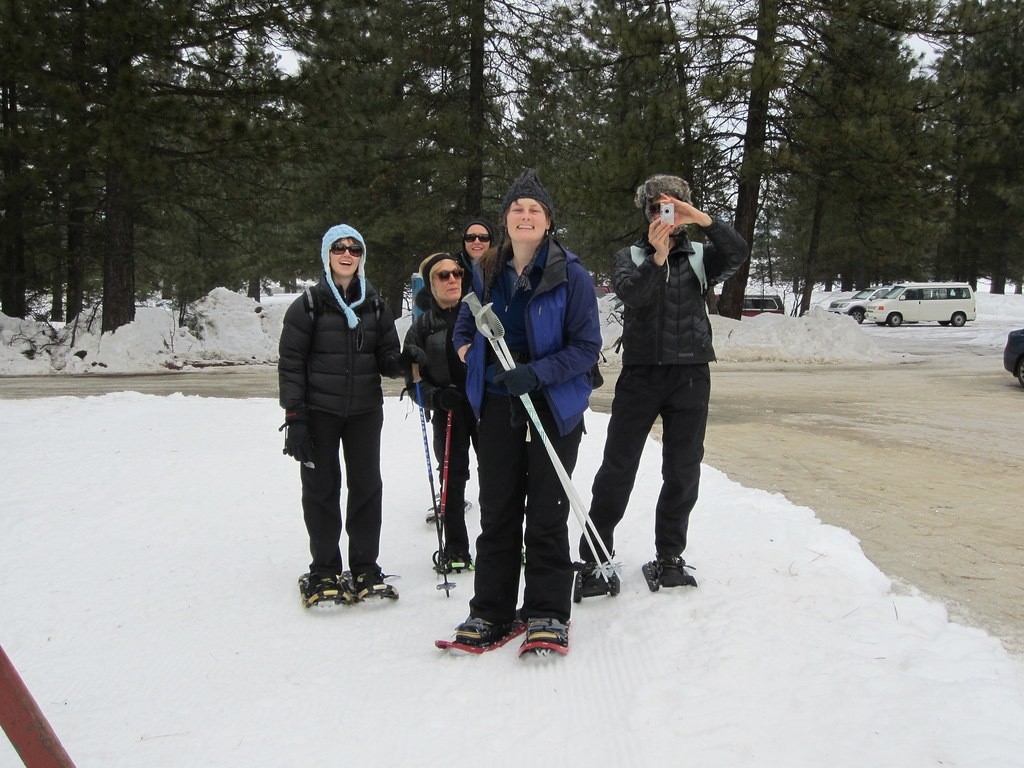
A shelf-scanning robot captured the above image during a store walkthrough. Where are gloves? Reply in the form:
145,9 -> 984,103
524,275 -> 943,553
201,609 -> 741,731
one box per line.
398,345 -> 426,370
434,388 -> 467,411
494,363 -> 538,397
285,406 -> 314,463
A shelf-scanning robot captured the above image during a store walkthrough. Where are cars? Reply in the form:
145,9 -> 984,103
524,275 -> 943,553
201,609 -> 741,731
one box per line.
1004,328 -> 1024,389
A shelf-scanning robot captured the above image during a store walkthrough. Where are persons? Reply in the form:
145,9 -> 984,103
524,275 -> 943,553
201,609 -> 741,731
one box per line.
279,224 -> 428,593
454,169 -> 602,642
580,174 -> 752,588
402,220 -> 527,573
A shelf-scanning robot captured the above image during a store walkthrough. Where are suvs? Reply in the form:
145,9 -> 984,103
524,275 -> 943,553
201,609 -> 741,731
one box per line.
827,286 -> 894,325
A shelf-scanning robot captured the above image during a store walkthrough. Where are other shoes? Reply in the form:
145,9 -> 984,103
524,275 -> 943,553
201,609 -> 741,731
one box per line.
445,551 -> 466,564
581,563 -> 603,587
660,560 -> 680,586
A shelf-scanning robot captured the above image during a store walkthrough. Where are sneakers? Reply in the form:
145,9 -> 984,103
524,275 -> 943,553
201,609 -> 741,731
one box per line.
354,572 -> 384,595
455,616 -> 517,645
526,617 -> 568,647
305,571 -> 343,600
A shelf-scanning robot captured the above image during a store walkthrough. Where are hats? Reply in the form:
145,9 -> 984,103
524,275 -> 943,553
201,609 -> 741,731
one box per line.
634,174 -> 692,209
322,223 -> 367,328
501,168 -> 557,221
462,216 -> 494,247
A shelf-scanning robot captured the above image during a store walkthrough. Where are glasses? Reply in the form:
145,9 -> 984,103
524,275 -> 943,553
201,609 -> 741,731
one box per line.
436,268 -> 466,282
331,242 -> 364,257
464,234 -> 492,243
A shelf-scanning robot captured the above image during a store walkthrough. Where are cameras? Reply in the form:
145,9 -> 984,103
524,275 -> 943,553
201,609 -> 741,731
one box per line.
660,202 -> 674,225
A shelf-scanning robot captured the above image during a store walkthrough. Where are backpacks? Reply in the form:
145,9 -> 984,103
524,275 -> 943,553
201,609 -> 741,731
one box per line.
565,262 -> 604,390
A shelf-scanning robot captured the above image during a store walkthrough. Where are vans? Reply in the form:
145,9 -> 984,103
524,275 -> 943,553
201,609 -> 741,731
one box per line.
715,294 -> 785,317
864,281 -> 977,328
